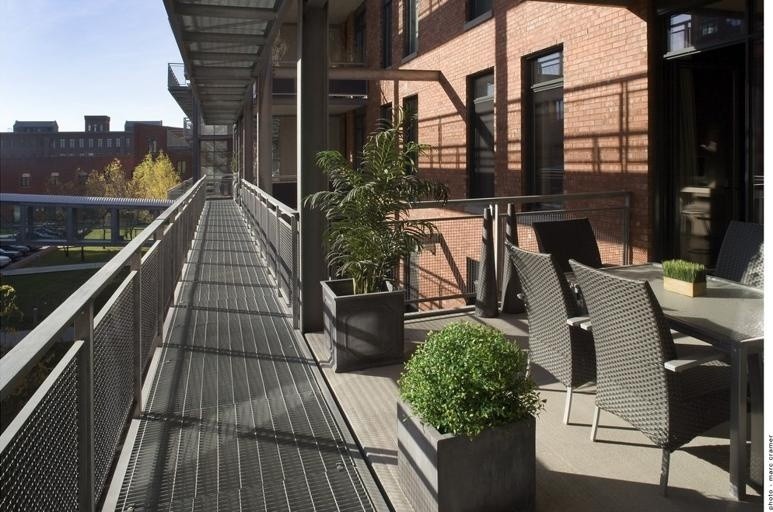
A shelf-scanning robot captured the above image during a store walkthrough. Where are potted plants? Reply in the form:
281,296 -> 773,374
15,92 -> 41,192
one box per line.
396,322 -> 548,512
303,103 -> 452,374
662,259 -> 706,298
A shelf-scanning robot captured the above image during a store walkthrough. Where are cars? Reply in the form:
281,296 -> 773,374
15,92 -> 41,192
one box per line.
0,223 -> 87,268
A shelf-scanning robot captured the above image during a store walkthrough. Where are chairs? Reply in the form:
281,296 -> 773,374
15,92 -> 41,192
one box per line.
504,239 -> 590,425
532,218 -> 602,339
714,219 -> 763,291
